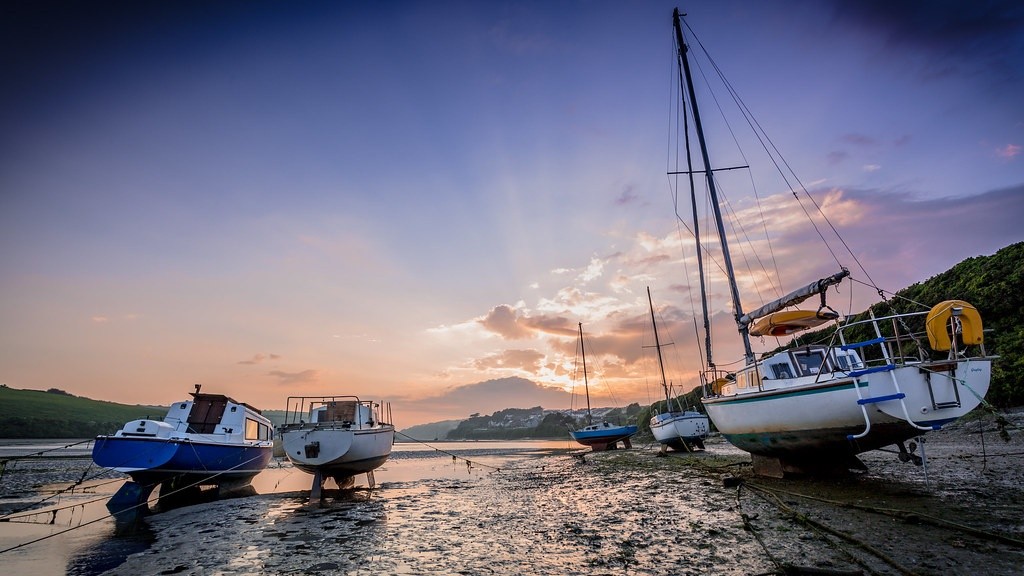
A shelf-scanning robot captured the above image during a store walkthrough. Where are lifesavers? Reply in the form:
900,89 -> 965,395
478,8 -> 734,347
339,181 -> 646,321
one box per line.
926,299 -> 984,351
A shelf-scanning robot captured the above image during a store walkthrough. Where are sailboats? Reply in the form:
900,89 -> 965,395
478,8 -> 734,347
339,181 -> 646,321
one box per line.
643,286 -> 710,444
568,322 -> 638,445
667,6 -> 1001,465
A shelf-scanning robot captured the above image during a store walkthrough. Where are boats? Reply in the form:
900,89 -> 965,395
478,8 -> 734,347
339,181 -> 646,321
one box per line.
92,384 -> 275,472
277,396 -> 394,477
